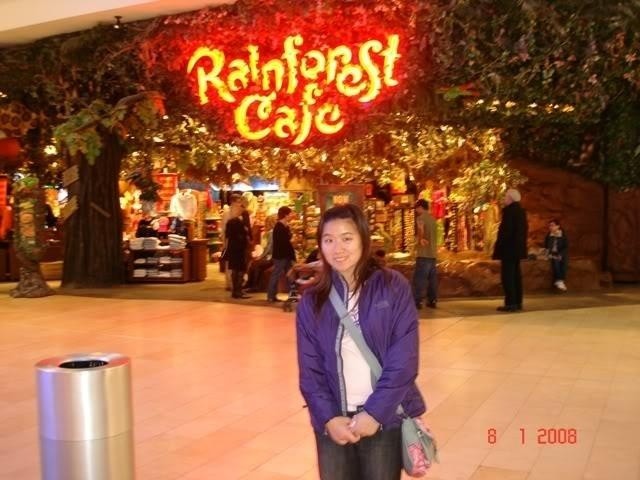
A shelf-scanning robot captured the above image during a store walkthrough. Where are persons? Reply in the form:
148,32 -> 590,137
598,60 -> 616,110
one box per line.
261,206 -> 302,305
170,183 -> 199,221
406,198 -> 439,308
542,219 -> 569,293
224,203 -> 251,299
375,249 -> 384,262
221,194 -> 245,290
492,189 -> 528,313
292,201 -> 428,480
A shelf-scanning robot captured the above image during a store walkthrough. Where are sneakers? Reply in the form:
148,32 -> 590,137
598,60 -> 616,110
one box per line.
416,299 -> 424,309
425,298 -> 437,307
554,280 -> 567,291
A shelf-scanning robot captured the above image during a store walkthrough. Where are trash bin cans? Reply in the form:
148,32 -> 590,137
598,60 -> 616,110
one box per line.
36,351 -> 133,479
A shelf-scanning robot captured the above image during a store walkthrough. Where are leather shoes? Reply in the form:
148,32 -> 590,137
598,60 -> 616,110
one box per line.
267,297 -> 283,302
497,305 -> 522,311
233,295 -> 251,299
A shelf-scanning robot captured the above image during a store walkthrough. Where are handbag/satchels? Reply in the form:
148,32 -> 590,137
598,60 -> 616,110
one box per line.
401,416 -> 438,477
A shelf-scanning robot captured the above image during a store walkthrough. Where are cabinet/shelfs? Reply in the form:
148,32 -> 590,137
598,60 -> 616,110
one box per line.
126,217 -> 194,283
